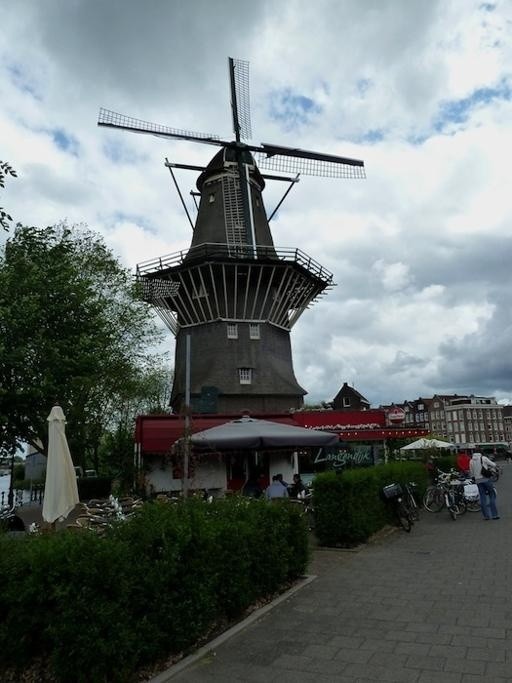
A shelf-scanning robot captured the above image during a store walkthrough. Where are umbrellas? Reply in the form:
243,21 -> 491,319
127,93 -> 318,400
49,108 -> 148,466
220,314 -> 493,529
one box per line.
41,405 -> 80,522
171,418 -> 339,464
430,438 -> 454,457
400,438 -> 432,450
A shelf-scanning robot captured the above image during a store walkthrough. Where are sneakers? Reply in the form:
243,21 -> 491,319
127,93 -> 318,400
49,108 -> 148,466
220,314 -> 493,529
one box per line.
486,517 -> 500,520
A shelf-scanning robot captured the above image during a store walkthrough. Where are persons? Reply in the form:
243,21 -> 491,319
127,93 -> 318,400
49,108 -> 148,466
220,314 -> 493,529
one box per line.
504,450 -> 512,464
246,473 -> 311,506
457,451 -> 471,473
470,447 -> 500,520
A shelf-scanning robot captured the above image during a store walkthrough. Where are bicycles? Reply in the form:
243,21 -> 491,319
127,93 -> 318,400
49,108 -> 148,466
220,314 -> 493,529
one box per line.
67,487 -> 215,538
381,466 -> 500,531
298,490 -> 317,533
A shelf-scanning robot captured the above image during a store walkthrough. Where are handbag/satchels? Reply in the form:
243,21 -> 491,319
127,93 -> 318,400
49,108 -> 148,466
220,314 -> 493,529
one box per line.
481,467 -> 493,478
464,484 -> 479,497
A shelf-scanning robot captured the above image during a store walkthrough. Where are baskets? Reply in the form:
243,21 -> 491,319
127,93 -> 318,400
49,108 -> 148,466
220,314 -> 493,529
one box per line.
383,482 -> 403,498
